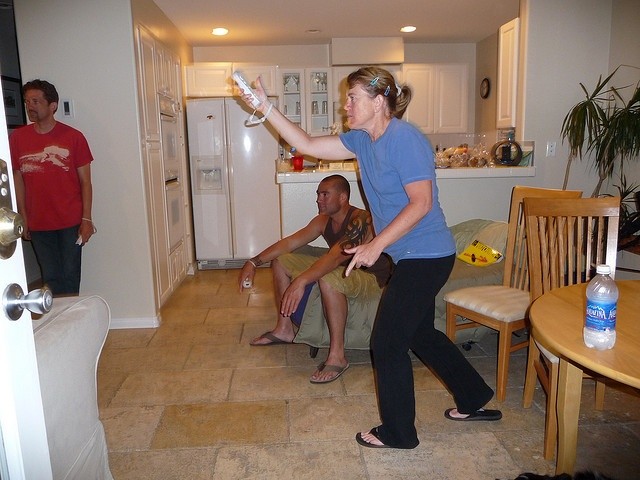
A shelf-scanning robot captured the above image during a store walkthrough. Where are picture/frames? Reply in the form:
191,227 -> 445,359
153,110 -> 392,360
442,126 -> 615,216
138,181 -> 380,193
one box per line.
0,74 -> 26,130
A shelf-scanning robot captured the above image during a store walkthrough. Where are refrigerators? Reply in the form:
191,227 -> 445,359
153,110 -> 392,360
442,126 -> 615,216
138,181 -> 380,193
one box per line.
184,96 -> 282,271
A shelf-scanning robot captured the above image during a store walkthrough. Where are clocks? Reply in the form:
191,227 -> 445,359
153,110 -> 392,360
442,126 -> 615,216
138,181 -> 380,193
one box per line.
480,78 -> 490,99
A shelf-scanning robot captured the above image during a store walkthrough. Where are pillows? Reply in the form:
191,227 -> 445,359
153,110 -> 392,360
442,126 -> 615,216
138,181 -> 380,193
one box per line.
448,218 -> 510,259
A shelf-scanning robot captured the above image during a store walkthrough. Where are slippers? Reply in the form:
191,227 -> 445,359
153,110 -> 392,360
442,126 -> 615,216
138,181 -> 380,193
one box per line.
310,361 -> 350,384
356,427 -> 392,448
249,331 -> 294,346
444,408 -> 502,421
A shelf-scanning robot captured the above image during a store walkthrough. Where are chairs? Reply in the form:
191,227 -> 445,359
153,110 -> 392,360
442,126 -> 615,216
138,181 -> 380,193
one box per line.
519,196 -> 622,461
442,185 -> 583,403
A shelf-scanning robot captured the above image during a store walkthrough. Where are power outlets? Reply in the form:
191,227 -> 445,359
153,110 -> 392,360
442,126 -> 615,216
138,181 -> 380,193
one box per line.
546,142 -> 556,157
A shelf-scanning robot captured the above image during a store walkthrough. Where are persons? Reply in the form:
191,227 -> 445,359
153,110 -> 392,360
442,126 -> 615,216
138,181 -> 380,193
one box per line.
236,65 -> 494,448
8,79 -> 93,296
238,174 -> 394,384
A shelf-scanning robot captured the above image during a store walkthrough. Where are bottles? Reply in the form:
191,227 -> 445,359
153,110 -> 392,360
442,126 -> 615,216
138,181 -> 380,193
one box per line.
582,264 -> 619,351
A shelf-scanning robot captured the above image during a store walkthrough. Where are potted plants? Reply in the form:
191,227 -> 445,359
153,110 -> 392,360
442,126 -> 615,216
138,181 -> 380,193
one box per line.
561,64 -> 640,270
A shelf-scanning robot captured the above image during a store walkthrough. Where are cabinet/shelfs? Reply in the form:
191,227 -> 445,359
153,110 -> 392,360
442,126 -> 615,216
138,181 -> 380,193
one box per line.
494,16 -> 518,130
136,25 -> 192,307
183,63 -> 277,98
281,63 -> 470,137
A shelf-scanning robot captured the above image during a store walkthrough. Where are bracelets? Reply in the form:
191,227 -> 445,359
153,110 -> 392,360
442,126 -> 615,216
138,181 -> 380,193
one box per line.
247,258 -> 258,268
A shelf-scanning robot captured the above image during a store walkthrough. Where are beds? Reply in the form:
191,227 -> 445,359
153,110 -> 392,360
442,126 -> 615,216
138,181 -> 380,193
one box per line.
290,217 -> 529,359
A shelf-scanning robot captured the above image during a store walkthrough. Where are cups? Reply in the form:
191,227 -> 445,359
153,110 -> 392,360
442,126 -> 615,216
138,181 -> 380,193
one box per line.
291,156 -> 305,171
275,158 -> 291,173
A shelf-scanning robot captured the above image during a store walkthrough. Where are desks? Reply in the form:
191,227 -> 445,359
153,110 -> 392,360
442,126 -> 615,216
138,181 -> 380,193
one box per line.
528,279 -> 640,476
